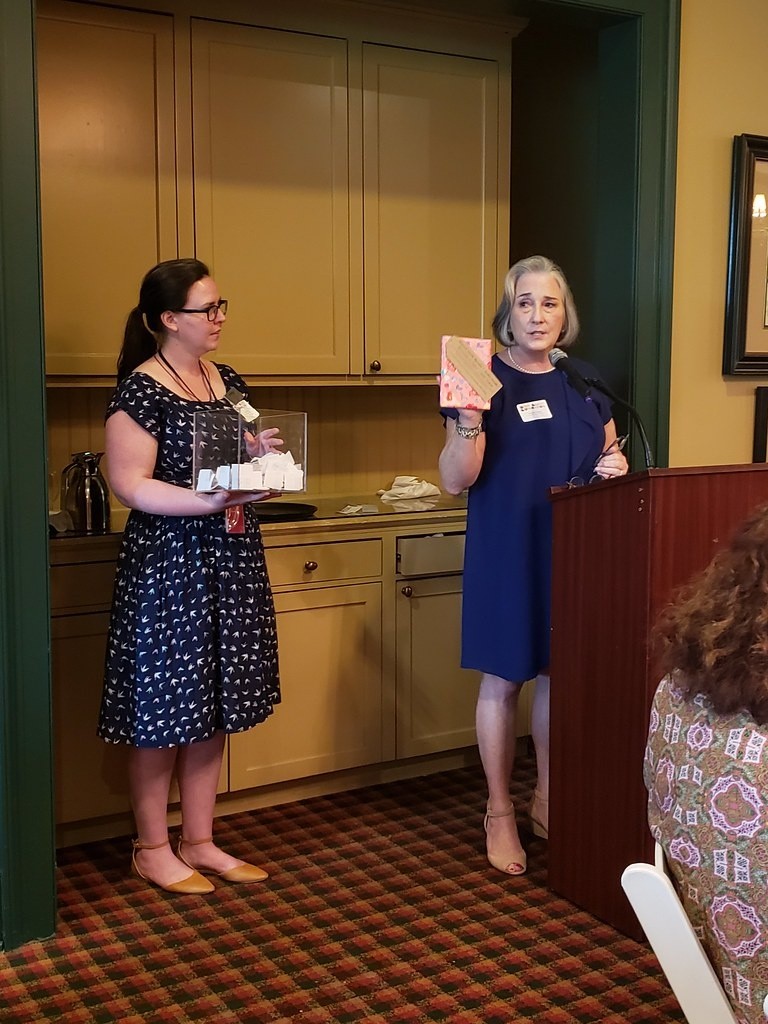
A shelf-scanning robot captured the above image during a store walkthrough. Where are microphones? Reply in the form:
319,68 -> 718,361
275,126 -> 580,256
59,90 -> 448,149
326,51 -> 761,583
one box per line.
549,347 -> 592,399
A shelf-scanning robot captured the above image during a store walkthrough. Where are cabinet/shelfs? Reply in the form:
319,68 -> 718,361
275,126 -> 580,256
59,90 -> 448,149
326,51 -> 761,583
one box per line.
50,509 -> 534,848
182,0 -> 532,386
29,0 -> 181,394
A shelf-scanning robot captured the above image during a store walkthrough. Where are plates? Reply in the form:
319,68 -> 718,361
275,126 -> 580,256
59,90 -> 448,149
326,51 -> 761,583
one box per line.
250,502 -> 318,520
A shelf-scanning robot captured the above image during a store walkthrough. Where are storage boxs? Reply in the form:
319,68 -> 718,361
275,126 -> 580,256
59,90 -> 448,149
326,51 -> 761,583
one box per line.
440,334 -> 493,410
192,407 -> 307,495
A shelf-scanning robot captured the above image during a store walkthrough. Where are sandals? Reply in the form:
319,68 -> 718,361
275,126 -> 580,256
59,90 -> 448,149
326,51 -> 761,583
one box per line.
176,835 -> 268,882
130,839 -> 215,894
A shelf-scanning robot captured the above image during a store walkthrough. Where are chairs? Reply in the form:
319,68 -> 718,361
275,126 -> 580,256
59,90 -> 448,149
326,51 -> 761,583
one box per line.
621,841 -> 768,1024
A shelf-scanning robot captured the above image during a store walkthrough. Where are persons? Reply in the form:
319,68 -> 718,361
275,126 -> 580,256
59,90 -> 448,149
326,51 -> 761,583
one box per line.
95,258 -> 285,894
643,505 -> 768,1024
437,256 -> 628,875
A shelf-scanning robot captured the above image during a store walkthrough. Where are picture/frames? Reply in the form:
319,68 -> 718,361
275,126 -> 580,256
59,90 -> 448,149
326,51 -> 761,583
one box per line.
721,133 -> 768,376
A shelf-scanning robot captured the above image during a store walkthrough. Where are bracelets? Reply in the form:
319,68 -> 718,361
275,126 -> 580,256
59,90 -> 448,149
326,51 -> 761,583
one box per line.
454,416 -> 483,439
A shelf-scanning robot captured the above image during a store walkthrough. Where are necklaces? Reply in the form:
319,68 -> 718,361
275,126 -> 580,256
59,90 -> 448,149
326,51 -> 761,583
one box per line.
507,346 -> 556,374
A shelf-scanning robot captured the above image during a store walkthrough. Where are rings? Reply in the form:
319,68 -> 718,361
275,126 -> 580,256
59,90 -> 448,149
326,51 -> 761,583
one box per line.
620,470 -> 623,476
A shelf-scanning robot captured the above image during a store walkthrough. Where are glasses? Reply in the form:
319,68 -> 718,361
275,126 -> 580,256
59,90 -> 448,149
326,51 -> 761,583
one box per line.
594,434 -> 629,466
565,473 -> 613,490
174,300 -> 228,322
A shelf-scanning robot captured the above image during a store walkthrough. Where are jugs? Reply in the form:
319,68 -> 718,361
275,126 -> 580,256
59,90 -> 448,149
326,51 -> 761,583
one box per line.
60,451 -> 110,536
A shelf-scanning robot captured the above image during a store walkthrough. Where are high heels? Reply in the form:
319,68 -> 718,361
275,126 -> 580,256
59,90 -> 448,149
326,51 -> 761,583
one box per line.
484,798 -> 526,875
531,788 -> 548,840
